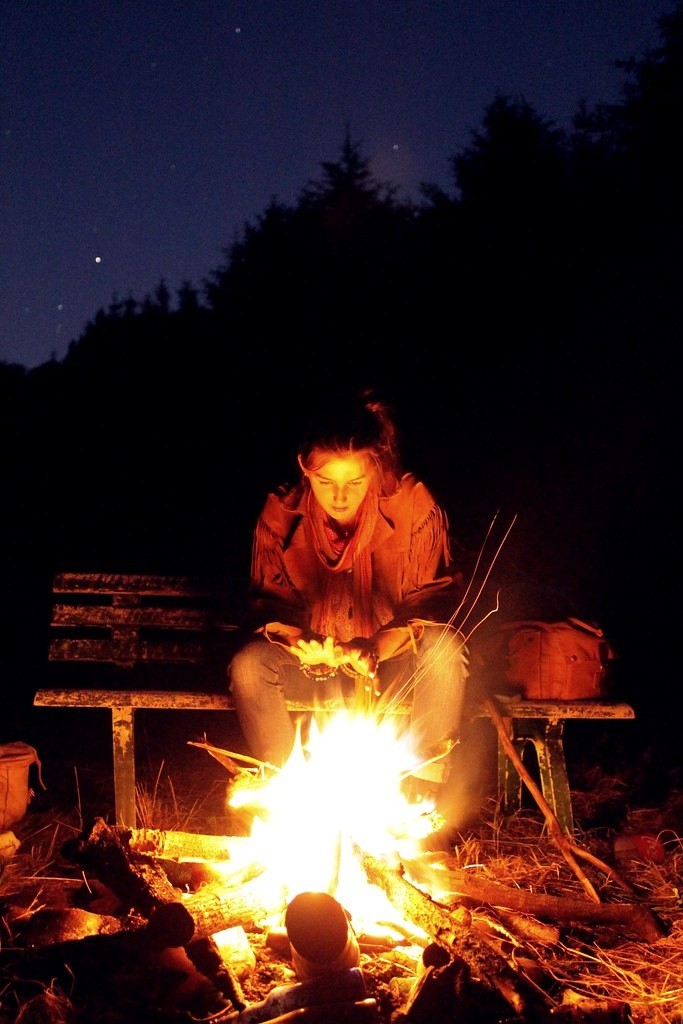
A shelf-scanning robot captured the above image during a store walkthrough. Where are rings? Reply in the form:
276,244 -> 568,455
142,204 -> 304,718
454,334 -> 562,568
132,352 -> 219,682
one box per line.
310,645 -> 323,653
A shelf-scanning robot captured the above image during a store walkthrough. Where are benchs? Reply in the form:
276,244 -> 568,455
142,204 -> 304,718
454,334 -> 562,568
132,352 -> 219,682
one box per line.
34,574 -> 633,845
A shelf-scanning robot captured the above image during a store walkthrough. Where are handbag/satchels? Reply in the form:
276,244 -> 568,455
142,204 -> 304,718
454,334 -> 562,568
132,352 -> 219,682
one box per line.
490,613 -> 622,702
0,742 -> 48,835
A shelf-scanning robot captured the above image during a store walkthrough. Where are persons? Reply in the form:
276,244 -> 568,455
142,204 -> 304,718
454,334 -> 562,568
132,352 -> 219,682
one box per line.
227,387 -> 468,803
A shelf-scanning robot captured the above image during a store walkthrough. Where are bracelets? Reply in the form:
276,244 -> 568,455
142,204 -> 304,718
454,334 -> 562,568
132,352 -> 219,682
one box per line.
300,663 -> 338,681
339,637 -> 380,679
